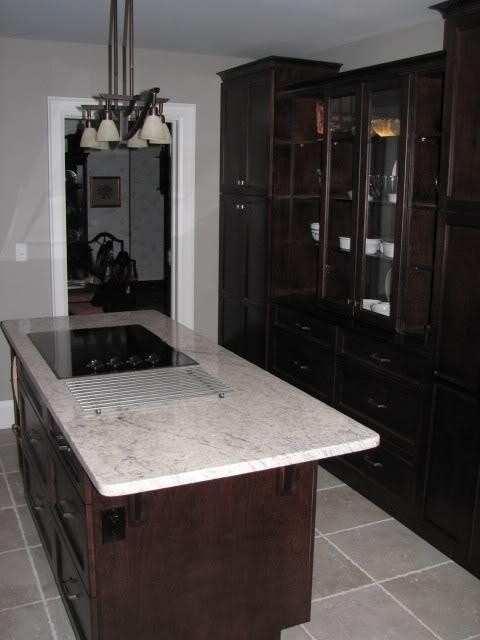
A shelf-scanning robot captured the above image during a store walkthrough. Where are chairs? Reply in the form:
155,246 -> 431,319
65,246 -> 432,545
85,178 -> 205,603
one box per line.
100,250 -> 138,311
86,231 -> 124,270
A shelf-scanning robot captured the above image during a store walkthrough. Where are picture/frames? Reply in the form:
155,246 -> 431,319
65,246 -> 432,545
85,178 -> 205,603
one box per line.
89,175 -> 122,208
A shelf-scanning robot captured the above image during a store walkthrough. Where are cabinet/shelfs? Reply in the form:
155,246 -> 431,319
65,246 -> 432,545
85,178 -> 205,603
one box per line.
9,348 -> 90,640
214,55 -> 345,481
345,1 -> 478,579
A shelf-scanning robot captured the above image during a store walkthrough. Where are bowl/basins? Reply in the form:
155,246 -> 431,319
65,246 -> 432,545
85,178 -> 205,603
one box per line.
366,238 -> 381,255
383,240 -> 394,258
338,237 -> 351,249
311,222 -> 319,242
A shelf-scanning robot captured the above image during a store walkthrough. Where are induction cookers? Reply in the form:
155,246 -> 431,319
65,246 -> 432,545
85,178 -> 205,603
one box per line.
26,323 -> 200,379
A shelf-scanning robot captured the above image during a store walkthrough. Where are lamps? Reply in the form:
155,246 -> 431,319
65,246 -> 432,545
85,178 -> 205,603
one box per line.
79,0 -> 172,151
